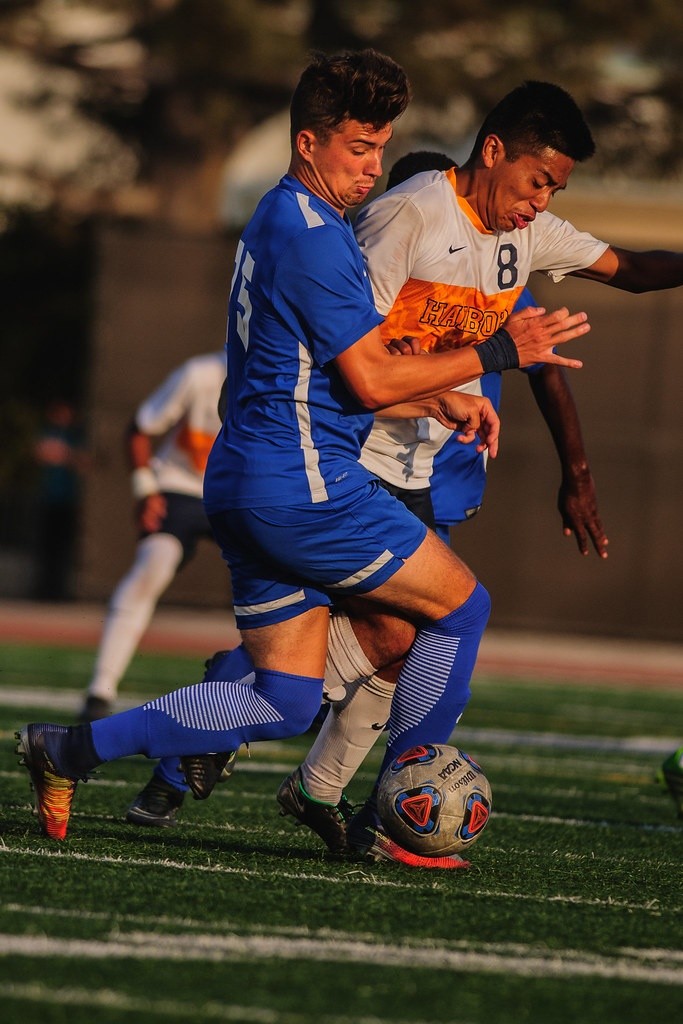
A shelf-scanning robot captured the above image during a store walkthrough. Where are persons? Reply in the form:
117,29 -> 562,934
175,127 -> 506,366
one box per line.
14,46 -> 683,868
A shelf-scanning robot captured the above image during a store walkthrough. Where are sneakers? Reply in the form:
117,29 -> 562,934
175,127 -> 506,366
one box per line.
277,763 -> 382,868
347,805 -> 470,870
177,742 -> 236,801
14,723 -> 98,844
125,773 -> 189,825
201,649 -> 251,782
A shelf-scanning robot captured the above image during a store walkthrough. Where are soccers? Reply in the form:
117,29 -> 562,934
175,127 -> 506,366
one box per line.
375,742 -> 494,859
